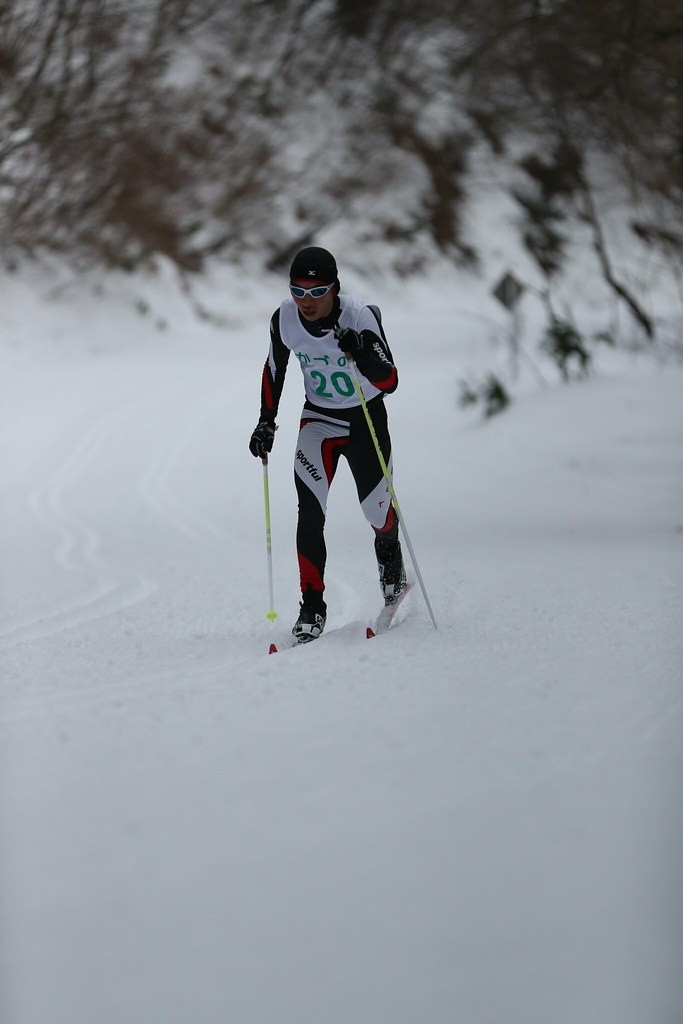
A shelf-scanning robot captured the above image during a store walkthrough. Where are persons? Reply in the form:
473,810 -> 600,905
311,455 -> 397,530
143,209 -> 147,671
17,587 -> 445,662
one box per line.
247,245 -> 407,641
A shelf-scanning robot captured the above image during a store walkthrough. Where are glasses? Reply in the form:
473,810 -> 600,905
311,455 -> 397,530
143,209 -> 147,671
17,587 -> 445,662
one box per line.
289,282 -> 334,298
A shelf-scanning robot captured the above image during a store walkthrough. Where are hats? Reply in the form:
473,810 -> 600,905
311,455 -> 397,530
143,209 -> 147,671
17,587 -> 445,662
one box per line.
290,247 -> 338,281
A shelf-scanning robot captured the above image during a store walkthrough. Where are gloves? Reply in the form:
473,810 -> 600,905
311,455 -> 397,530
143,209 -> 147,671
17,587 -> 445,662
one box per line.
334,327 -> 362,359
249,416 -> 277,459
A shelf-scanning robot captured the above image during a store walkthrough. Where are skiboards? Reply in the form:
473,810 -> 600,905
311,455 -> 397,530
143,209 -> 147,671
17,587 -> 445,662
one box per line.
267,578 -> 418,655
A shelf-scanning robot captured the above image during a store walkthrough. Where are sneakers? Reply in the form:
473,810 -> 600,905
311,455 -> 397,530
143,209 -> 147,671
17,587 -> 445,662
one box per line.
373,537 -> 406,599
292,590 -> 327,639
